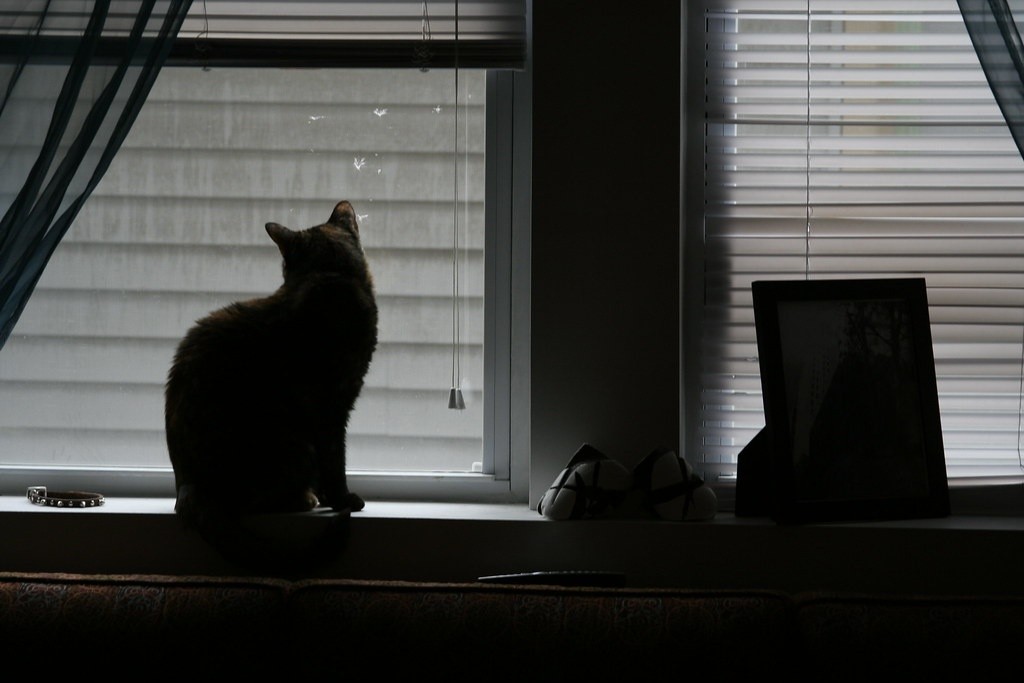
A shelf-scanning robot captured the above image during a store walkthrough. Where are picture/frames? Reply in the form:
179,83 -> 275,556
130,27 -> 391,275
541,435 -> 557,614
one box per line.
753,278 -> 950,523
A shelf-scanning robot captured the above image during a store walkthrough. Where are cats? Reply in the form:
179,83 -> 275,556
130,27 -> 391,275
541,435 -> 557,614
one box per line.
166,201 -> 376,581
